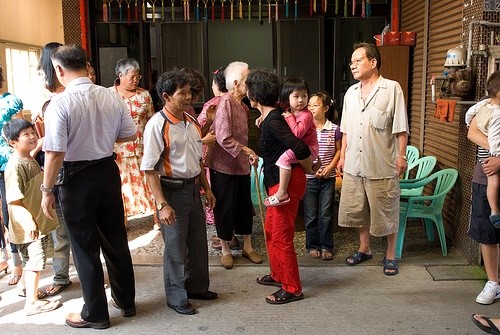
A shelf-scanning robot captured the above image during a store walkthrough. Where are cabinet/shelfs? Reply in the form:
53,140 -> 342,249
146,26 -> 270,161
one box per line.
376,46 -> 409,114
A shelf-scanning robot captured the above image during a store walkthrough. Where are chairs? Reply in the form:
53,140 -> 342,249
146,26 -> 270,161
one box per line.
250,156 -> 264,216
381,144 -> 458,258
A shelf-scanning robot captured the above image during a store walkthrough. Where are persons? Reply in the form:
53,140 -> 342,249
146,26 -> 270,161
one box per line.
0,42 -> 97,315
299,92 -> 341,259
465,71 -> 500,335
246,69 -> 318,304
337,43 -> 410,275
139,68 -> 218,315
41,44 -> 138,329
107,58 -> 162,231
196,61 -> 262,269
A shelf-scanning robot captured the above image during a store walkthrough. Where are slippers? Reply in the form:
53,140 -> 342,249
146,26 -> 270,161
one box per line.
262,195 -> 290,207
265,288 -> 304,304
25,299 -> 61,316
309,249 -> 320,259
17,288 -> 45,298
382,255 -> 398,275
9,274 -> 22,285
46,281 -> 72,296
345,251 -> 373,265
256,275 -> 282,286
471,314 -> 500,335
320,250 -> 333,260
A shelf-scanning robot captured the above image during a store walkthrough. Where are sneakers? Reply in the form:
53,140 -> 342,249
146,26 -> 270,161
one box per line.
110,297 -> 136,316
66,314 -> 110,329
476,280 -> 500,305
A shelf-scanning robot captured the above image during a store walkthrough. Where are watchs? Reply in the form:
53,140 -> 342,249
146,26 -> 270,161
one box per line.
396,154 -> 408,160
157,201 -> 168,210
40,184 -> 54,192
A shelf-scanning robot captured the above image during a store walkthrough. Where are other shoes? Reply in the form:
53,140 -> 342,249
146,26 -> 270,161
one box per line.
490,214 -> 500,229
221,252 -> 234,269
187,291 -> 217,300
242,248 -> 261,264
211,234 -> 239,250
167,302 -> 195,314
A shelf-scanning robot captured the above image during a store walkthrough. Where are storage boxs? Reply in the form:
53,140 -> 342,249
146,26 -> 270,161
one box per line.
375,31 -> 417,47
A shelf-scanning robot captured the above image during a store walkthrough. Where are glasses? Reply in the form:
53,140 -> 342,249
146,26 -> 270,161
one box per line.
349,56 -> 374,66
120,74 -> 142,79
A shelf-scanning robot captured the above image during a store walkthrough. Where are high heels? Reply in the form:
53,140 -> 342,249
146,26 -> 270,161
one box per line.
0,265 -> 9,273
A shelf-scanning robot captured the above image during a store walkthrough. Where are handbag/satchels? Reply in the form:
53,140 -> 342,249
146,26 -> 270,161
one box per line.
202,103 -> 218,137
53,153 -> 117,186
